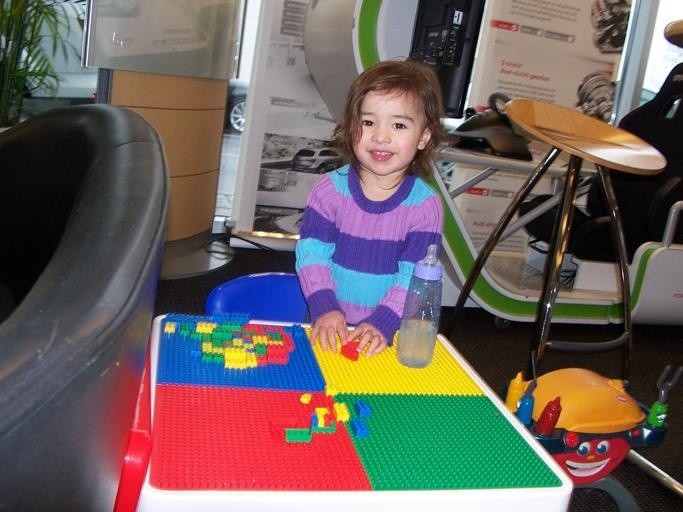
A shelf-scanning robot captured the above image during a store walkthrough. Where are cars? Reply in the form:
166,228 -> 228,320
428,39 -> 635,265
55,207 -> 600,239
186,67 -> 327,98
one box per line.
0,1 -> 97,105
225,76 -> 249,135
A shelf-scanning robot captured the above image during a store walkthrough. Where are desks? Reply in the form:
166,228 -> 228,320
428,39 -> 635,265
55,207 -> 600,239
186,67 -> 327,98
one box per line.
113,312 -> 573,512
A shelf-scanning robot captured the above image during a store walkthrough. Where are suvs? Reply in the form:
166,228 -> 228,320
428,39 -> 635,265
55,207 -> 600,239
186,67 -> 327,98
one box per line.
291,148 -> 343,175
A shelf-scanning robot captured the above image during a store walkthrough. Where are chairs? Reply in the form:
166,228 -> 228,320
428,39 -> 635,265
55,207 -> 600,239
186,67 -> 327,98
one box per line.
0,103 -> 169,511
202,269 -> 311,325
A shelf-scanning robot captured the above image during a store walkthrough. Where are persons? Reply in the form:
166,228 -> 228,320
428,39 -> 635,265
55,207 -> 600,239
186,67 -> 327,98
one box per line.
292,60 -> 447,356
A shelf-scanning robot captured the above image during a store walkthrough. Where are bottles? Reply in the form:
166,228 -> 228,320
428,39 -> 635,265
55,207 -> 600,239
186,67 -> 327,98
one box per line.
395,243 -> 445,367
534,395 -> 563,436
503,370 -> 525,415
514,387 -> 535,425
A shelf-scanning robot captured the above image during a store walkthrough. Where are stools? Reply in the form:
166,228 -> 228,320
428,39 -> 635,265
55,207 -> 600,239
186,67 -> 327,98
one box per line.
447,95 -> 665,387
